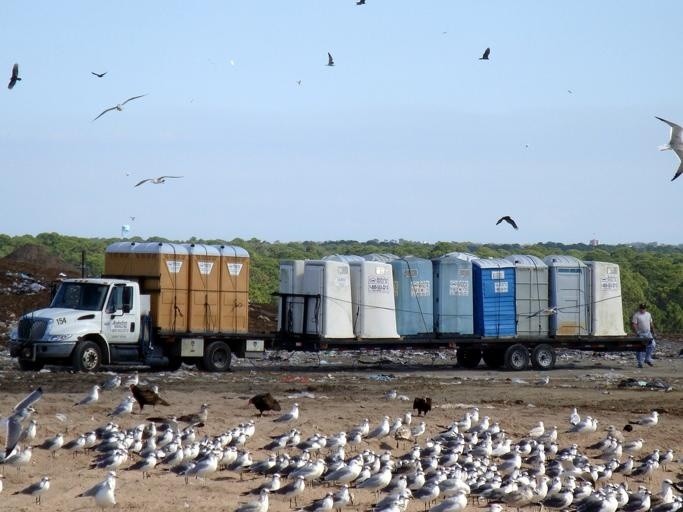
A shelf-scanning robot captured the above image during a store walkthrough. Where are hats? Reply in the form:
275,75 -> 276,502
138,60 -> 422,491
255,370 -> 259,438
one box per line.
639,303 -> 646,308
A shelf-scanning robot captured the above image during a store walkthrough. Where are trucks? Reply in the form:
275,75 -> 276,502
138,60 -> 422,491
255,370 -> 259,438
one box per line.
9,275 -> 276,373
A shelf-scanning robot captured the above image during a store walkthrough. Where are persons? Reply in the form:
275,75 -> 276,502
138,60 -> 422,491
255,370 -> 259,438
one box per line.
630,302 -> 656,368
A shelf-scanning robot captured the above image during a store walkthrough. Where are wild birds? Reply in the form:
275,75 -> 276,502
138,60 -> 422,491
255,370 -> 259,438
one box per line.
136,175 -> 182,186
8,63 -> 21,89
323,52 -> 335,66
478,47 -> 490,59
654,116 -> 683,181
0,370 -> 683,512
526,305 -> 565,319
356,0 -> 366,5
496,216 -> 517,228
91,72 -> 108,77
94,94 -> 145,121
